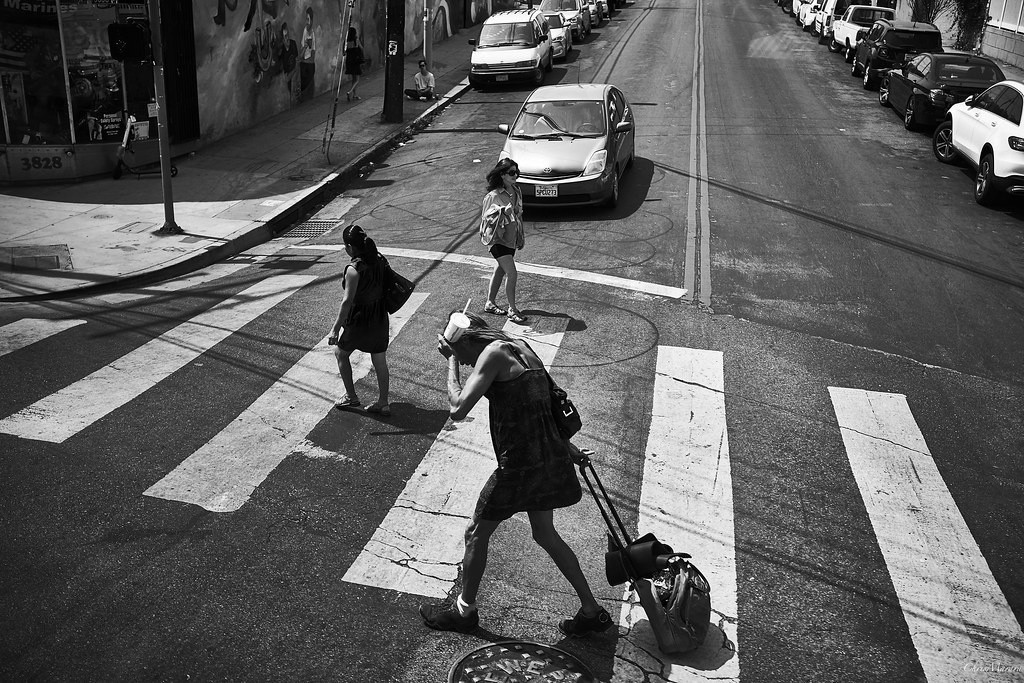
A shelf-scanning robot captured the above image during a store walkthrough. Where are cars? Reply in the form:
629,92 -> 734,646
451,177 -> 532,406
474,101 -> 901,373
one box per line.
931,78 -> 1024,209
588,0 -> 627,29
542,11 -> 572,63
496,83 -> 635,213
879,52 -> 1007,133
773,0 -> 877,46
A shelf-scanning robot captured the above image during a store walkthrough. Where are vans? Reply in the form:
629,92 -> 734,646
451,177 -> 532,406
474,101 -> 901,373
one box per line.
538,0 -> 591,45
468,8 -> 554,92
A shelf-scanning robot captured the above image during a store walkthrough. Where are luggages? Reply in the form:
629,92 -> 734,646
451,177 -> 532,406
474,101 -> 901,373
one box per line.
578,458 -> 711,653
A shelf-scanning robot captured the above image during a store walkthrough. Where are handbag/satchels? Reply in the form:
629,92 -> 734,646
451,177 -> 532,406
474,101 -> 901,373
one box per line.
546,370 -> 582,439
378,253 -> 415,315
351,39 -> 364,64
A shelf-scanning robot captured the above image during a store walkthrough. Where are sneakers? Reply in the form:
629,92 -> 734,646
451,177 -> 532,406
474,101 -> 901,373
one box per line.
418,588 -> 479,633
559,606 -> 614,638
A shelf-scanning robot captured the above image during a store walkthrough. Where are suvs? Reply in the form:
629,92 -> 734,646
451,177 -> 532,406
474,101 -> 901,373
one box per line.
851,18 -> 944,91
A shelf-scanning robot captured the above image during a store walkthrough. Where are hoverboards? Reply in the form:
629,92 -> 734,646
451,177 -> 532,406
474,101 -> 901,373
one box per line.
113,107 -> 179,180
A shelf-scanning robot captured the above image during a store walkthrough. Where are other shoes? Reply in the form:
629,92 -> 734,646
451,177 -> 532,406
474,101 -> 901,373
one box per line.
354,96 -> 361,99
347,92 -> 350,101
420,96 -> 427,101
407,96 -> 414,100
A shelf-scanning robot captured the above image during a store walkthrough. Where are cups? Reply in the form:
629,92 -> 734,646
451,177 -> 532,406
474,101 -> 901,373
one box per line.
441,313 -> 470,347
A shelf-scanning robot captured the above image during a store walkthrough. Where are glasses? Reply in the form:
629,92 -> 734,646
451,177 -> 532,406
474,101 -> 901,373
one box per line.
505,169 -> 519,176
419,65 -> 425,67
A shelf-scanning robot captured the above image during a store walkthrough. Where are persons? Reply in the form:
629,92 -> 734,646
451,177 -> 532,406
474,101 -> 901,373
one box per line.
418,310 -> 619,641
404,60 -> 436,102
329,225 -> 390,415
342,28 -> 364,101
575,106 -> 601,131
483,157 -> 528,322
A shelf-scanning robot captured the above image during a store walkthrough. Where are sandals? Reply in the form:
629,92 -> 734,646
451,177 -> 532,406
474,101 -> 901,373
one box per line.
364,401 -> 390,415
335,395 -> 360,407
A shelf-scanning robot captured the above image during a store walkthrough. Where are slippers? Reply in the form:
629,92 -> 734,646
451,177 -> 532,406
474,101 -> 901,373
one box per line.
485,306 -> 506,314
507,309 -> 527,323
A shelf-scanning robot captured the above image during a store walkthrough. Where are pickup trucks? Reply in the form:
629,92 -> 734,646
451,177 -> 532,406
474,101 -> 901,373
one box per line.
828,4 -> 896,64
